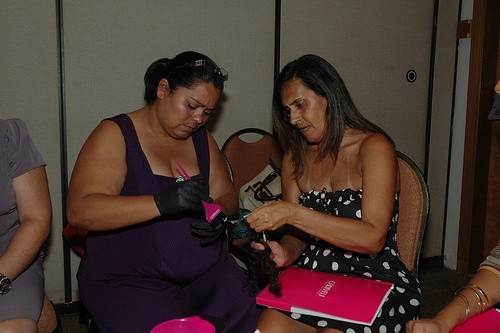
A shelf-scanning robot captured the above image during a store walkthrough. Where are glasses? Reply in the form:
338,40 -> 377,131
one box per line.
166,59 -> 229,82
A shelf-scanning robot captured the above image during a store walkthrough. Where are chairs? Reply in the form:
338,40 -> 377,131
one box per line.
222,128 -> 282,191
396,151 -> 429,279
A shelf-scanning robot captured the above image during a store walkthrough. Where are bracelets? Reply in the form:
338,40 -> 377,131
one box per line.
454,283 -> 489,320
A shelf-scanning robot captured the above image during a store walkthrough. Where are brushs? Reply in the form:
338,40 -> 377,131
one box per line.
175,160 -> 228,229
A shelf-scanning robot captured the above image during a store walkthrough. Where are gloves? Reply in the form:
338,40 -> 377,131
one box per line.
190,212 -> 231,247
154,174 -> 214,216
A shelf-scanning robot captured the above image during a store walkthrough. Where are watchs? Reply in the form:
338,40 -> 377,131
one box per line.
0,273 -> 12,295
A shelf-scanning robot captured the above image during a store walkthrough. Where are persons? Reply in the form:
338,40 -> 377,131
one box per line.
67,51 -> 262,333
247,54 -> 426,333
405,240 -> 500,333
0,118 -> 53,333
